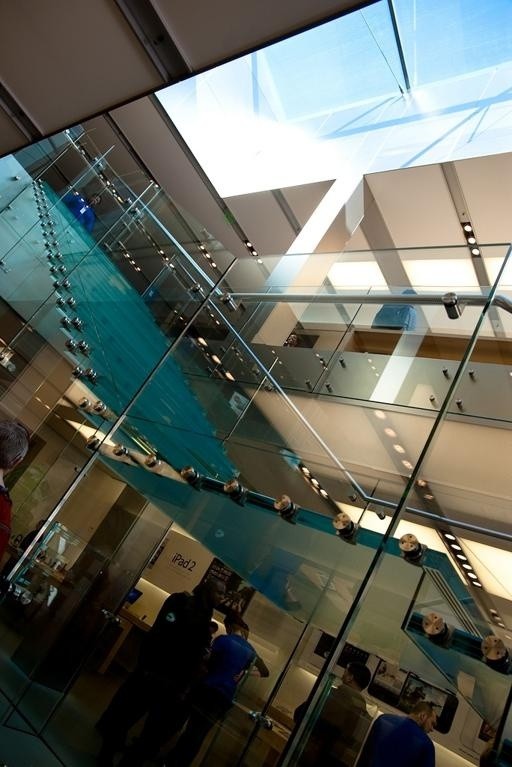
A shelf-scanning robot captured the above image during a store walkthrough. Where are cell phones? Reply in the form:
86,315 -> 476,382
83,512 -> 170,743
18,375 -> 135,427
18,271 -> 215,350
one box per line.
310,632 -> 458,733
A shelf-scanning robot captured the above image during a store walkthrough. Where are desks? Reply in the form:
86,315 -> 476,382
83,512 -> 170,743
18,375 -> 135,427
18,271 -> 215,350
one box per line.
10,557 -> 80,596
95,605 -> 154,679
187,692 -> 292,765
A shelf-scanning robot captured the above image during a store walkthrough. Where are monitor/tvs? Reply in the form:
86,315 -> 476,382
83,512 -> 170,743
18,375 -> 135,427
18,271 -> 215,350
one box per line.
127,587 -> 143,604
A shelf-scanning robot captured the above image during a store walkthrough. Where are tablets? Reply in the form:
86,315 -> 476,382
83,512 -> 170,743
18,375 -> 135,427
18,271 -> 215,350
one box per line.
192,557 -> 258,619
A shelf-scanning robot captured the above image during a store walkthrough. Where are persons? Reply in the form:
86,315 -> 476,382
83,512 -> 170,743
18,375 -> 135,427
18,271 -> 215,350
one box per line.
378,289 -> 417,328
0,519 -> 47,590
117,611 -> 270,766
1,419 -> 31,560
293,661 -> 371,765
94,575 -> 228,758
62,191 -> 104,231
357,700 -> 439,766
285,334 -> 299,345
